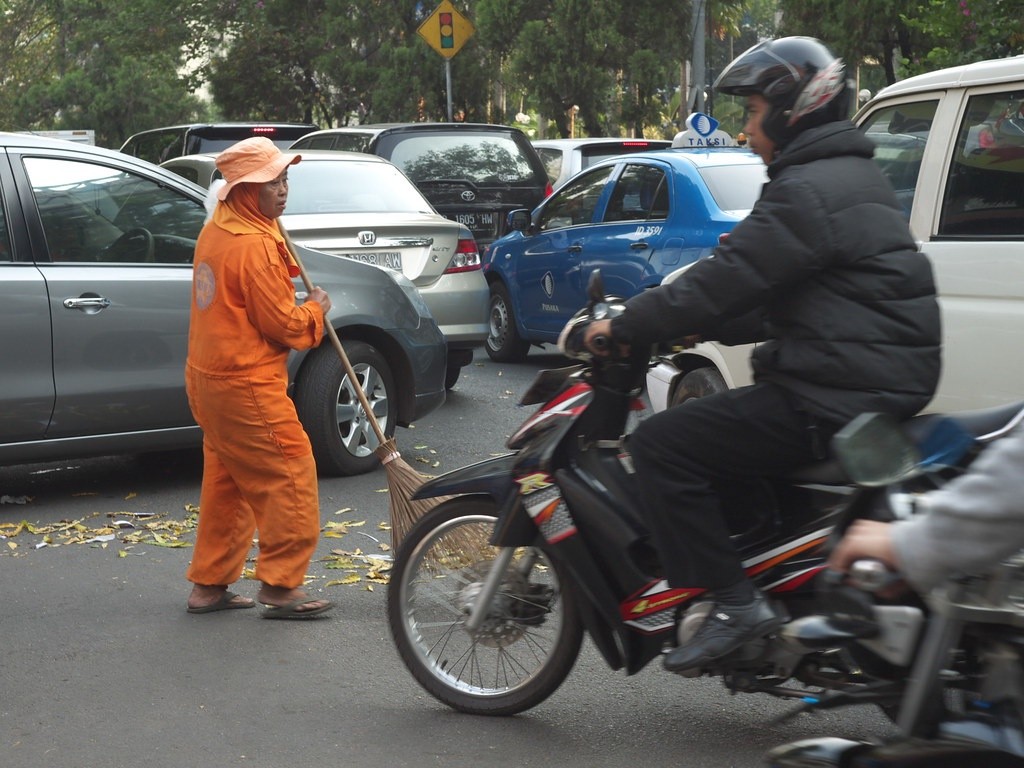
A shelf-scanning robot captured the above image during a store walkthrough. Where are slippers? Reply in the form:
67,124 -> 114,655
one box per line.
263,591 -> 334,620
186,592 -> 256,613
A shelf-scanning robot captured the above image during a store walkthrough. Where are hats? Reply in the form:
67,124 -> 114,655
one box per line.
216,136 -> 302,200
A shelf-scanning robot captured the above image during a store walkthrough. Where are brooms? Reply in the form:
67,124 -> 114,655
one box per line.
276,214 -> 533,577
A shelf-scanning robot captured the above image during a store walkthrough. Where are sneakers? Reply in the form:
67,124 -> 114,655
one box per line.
664,591 -> 791,677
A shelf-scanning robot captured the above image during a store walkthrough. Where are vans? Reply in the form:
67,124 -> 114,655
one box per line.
2,133 -> 450,484
831,54 -> 1024,424
291,121 -> 558,261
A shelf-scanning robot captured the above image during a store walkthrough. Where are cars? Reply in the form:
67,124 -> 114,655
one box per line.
478,120 -> 805,364
152,146 -> 492,399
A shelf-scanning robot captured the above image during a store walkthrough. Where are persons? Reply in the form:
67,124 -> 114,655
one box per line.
184,135 -> 335,618
826,401 -> 1024,768
583,34 -> 945,673
41,193 -> 97,262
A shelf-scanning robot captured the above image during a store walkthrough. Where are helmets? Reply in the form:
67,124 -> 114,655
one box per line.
713,34 -> 853,143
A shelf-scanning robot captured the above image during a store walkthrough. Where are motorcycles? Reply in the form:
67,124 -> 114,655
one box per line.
383,257 -> 1024,750
